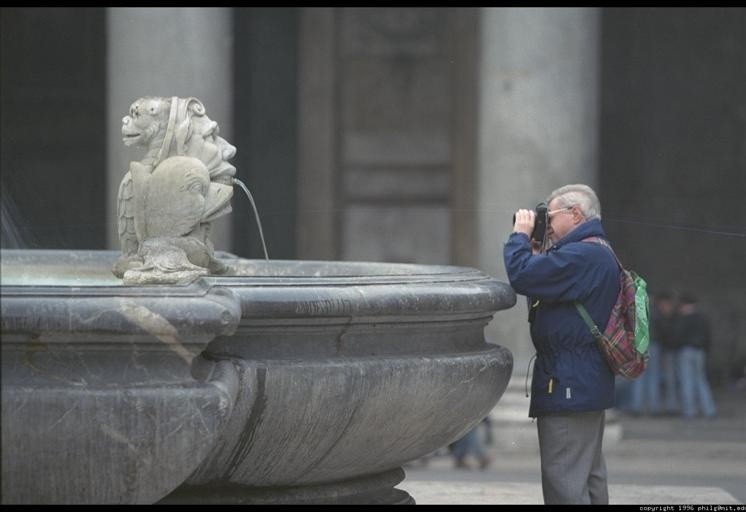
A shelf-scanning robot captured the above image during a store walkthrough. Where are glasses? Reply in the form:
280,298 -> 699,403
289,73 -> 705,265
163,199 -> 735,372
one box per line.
547,207 -> 575,216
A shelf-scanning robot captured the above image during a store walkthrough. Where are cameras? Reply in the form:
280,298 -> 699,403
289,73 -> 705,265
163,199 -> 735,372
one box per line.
513,201 -> 547,241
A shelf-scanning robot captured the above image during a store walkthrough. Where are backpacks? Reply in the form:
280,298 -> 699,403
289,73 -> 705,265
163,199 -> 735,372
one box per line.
573,237 -> 651,379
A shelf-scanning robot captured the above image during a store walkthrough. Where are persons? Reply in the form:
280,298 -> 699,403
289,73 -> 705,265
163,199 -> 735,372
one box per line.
504,185 -> 621,505
627,294 -> 718,418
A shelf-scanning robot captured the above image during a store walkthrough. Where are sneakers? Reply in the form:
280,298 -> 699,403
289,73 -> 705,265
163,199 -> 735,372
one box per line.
455,454 -> 491,473
